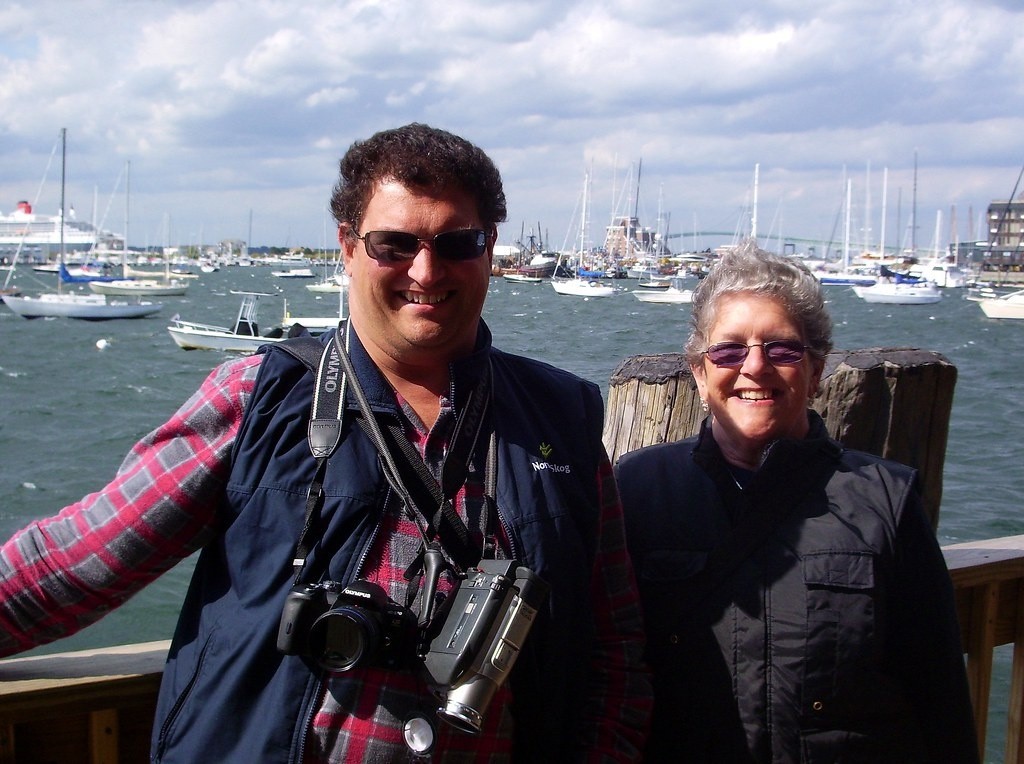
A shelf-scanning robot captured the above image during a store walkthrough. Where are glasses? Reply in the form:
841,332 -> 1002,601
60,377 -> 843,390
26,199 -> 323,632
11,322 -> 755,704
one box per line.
700,338 -> 814,367
351,223 -> 496,261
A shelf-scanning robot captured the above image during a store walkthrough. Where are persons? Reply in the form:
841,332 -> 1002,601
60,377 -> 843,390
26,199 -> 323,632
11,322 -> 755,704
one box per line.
617,235 -> 981,763
1,126 -> 654,764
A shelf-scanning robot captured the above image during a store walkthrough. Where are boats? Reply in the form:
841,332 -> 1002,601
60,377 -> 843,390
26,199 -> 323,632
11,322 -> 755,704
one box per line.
281,279 -> 347,336
166,288 -> 289,351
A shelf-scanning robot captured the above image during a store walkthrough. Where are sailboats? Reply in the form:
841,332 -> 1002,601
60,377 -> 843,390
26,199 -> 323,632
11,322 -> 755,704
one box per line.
0,124 -> 350,320
487,148 -> 1024,319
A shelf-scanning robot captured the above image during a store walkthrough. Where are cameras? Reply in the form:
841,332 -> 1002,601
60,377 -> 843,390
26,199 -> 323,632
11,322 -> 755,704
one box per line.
276,582 -> 419,675
417,558 -> 552,734
